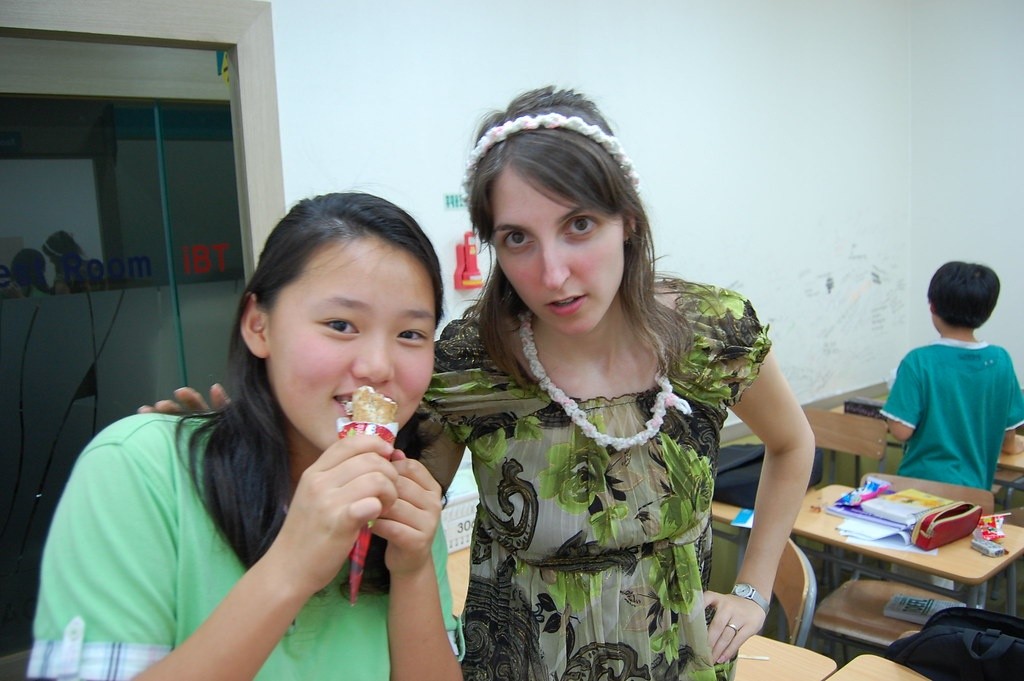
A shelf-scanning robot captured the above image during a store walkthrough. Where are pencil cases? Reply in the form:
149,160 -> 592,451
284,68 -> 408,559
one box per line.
911,501 -> 983,551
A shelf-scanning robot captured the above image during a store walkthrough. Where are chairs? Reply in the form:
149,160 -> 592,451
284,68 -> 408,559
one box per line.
804,406 -> 888,586
813,472 -> 996,663
772,538 -> 817,648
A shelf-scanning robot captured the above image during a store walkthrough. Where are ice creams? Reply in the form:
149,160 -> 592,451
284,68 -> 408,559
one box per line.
350,388 -> 397,424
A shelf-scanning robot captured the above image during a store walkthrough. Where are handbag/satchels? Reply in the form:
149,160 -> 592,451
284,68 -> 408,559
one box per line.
882,606 -> 1024,681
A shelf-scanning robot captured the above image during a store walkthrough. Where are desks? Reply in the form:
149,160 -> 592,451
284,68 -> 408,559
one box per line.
826,654 -> 932,681
712,487 -> 817,577
790,483 -> 1024,617
827,404 -> 905,489
735,635 -> 838,681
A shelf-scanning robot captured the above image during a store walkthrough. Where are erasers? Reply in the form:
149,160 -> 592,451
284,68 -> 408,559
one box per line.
971,538 -> 1006,558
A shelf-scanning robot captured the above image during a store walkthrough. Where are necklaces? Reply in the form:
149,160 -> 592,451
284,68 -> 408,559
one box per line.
520,310 -> 692,452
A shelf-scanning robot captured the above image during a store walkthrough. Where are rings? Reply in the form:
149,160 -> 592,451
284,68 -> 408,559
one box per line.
726,623 -> 738,635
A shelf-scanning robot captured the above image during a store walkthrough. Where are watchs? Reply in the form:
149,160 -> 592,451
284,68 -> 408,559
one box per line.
731,583 -> 770,615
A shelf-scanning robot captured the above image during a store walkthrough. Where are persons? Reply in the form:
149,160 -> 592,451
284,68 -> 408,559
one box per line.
879,260 -> 1024,591
136,86 -> 816,681
0,230 -> 102,299
25,192 -> 465,681
